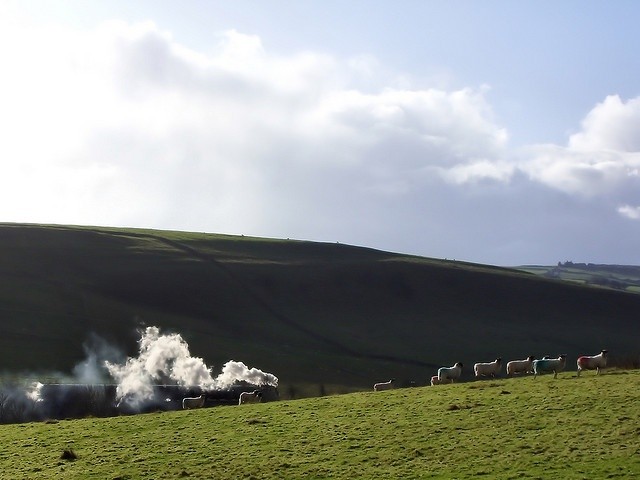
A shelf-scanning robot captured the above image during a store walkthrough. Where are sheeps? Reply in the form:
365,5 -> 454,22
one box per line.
374,377 -> 397,392
535,352 -> 568,380
506,354 -> 535,379
253,393 -> 262,403
473,356 -> 503,381
431,375 -> 451,385
182,393 -> 207,411
574,349 -> 609,377
531,354 -> 550,376
238,389 -> 260,405
438,362 -> 464,385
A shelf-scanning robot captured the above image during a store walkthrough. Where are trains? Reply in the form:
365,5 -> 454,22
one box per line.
1,380 -> 281,425
